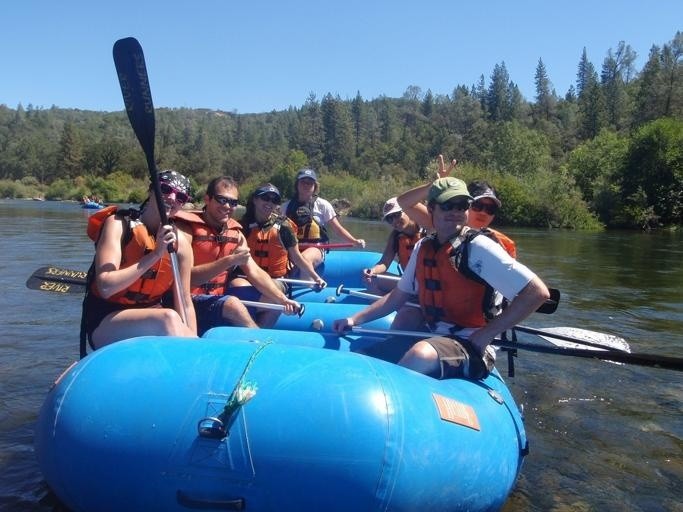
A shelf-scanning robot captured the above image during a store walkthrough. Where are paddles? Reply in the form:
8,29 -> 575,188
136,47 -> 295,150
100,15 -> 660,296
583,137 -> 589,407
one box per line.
111,35 -> 187,328
348,327 -> 683,373
26,266 -> 305,318
370,269 -> 561,316
336,283 -> 632,366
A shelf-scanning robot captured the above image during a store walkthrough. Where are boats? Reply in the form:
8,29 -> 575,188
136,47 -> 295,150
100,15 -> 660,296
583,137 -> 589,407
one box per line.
34,250 -> 530,508
82,202 -> 104,209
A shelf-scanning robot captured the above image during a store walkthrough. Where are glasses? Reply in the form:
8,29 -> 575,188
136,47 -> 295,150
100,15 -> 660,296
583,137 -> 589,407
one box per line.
260,194 -> 280,205
471,200 -> 497,216
160,182 -> 188,203
210,192 -> 239,207
434,199 -> 470,211
385,210 -> 403,224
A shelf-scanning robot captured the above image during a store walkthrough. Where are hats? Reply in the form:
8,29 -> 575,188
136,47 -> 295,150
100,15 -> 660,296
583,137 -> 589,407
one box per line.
427,177 -> 476,205
295,168 -> 317,182
468,187 -> 502,209
381,197 -> 403,223
149,169 -> 191,196
255,183 -> 281,199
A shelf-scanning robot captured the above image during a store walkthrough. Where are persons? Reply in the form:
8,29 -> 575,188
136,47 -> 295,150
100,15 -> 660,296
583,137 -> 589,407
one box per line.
396,155 -> 517,258
281,169 -> 366,267
231,183 -> 327,294
81,195 -> 104,209
334,177 -> 550,379
363,196 -> 427,284
80,169 -> 197,358
175,176 -> 299,337
